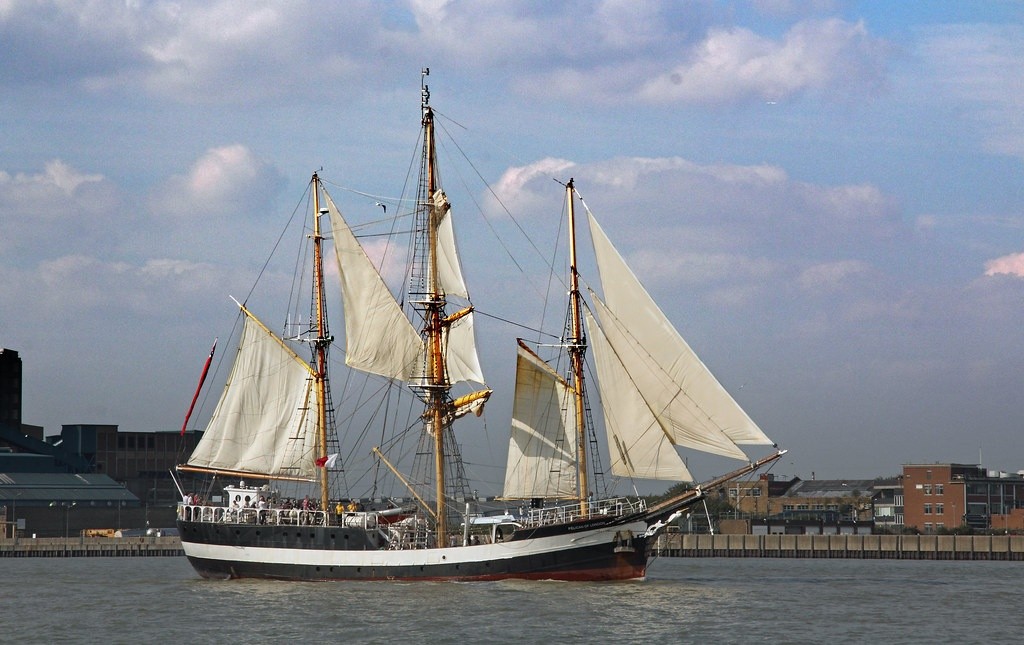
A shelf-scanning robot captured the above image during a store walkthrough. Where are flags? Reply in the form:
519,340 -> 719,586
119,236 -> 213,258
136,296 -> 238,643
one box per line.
181,337 -> 217,435
316,453 -> 338,468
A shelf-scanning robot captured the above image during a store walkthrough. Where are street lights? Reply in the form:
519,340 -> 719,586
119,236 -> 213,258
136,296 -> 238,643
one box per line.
145,488 -> 156,536
12,492 -> 22,537
119,490 -> 128,528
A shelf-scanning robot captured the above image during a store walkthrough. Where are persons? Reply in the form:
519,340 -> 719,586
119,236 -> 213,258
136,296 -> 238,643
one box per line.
450,534 -> 480,546
242,501 -> 256,512
302,495 -> 318,510
231,500 -> 239,515
589,492 -> 594,514
347,501 -> 357,512
336,502 -> 344,526
258,497 -> 267,525
183,493 -> 199,505
283,497 -> 293,510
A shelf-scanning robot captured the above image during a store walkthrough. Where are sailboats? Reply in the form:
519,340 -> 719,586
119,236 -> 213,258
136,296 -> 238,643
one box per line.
167,69 -> 787,583
